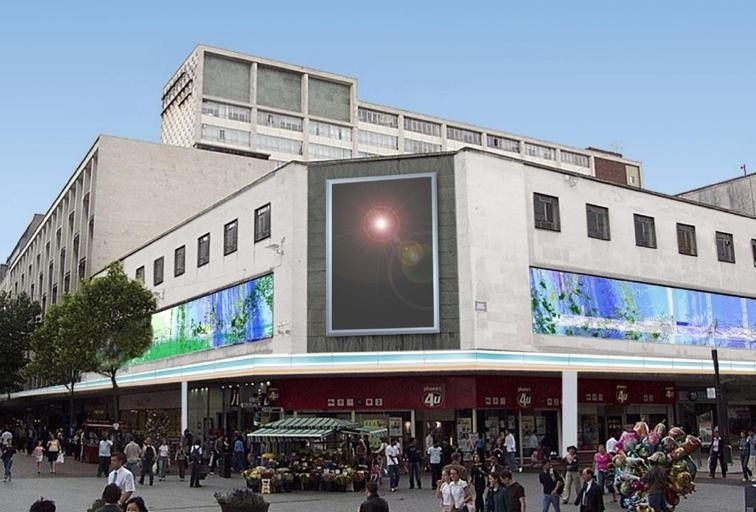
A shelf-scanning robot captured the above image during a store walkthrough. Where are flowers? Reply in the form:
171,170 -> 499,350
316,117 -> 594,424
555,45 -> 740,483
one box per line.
241,447 -> 367,492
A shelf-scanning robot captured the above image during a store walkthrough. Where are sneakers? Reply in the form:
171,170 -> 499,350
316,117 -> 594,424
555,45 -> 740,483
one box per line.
137,477 -> 202,488
389,486 -> 438,492
707,472 -> 756,483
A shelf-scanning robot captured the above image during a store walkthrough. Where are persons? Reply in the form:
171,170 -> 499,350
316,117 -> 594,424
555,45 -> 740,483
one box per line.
342,427 -> 617,512
123,428 -> 265,488
29,497 -> 56,512
2,427 -> 113,484
96,452 -> 148,512
710,426 -> 756,486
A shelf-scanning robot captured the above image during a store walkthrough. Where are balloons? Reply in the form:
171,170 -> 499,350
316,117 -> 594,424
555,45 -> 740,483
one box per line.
605,420 -> 703,512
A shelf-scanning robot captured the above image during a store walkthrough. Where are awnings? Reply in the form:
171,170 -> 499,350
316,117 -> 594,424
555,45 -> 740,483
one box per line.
246,417 -> 337,442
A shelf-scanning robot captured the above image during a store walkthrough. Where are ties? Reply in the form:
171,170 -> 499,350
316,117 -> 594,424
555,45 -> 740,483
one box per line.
112,472 -> 117,484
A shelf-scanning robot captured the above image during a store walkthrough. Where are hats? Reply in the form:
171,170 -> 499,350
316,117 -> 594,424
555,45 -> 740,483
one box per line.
365,480 -> 379,493
441,460 -> 466,473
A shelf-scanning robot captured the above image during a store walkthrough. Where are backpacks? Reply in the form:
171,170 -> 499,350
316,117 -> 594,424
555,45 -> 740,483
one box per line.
190,449 -> 201,461
144,443 -> 154,461
